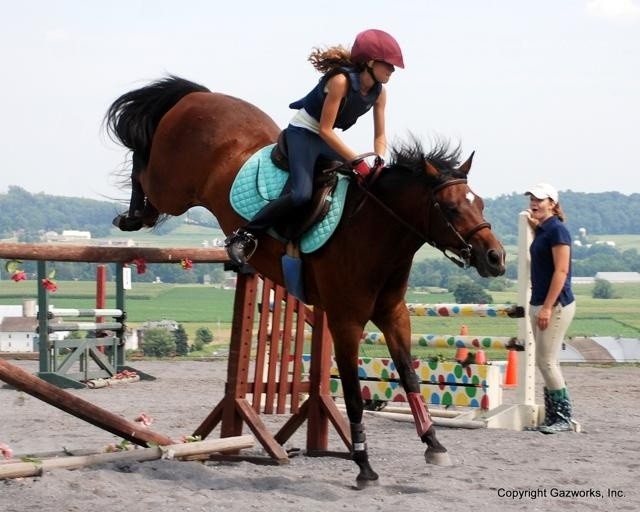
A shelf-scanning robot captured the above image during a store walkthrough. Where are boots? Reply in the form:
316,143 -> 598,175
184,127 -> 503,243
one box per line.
541,400 -> 573,434
525,387 -> 555,431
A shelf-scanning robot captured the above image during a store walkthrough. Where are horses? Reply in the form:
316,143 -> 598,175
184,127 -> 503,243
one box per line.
102,72 -> 510,490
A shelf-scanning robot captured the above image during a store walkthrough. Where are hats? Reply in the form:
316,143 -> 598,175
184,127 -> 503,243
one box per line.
524,184 -> 558,203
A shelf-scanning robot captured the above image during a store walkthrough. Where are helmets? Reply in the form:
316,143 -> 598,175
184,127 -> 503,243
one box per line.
349,29 -> 405,69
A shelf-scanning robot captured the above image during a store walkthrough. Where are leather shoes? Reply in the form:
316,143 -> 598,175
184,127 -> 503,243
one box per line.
225,230 -> 249,268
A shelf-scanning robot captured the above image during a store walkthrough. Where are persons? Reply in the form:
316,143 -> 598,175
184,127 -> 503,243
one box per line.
524,186 -> 578,434
226,30 -> 404,264
